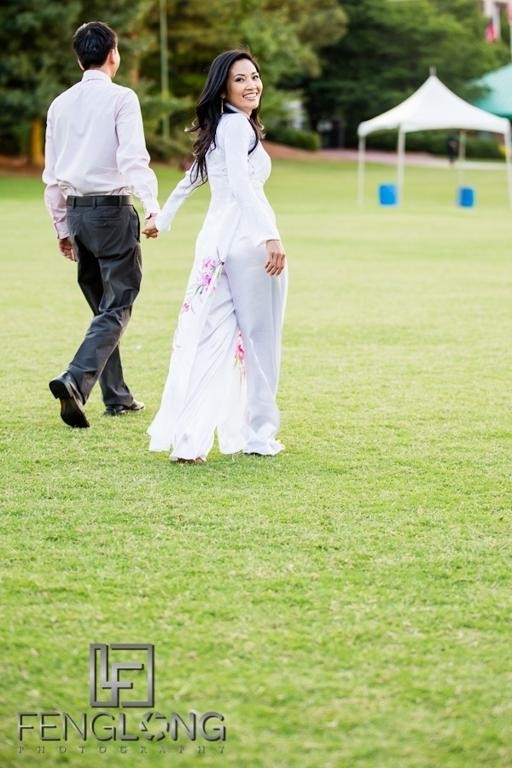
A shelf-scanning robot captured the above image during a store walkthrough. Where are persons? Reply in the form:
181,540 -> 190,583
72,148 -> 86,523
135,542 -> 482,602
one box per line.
41,21 -> 160,427
140,48 -> 288,463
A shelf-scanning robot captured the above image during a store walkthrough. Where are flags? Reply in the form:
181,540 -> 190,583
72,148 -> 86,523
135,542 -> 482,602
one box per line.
478,0 -> 504,46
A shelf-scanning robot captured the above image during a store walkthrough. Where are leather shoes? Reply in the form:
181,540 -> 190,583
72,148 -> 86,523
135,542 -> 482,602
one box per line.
104,397 -> 146,415
48,371 -> 91,428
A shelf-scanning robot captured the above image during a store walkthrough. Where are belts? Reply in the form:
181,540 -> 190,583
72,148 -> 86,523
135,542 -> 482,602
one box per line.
66,194 -> 134,207
